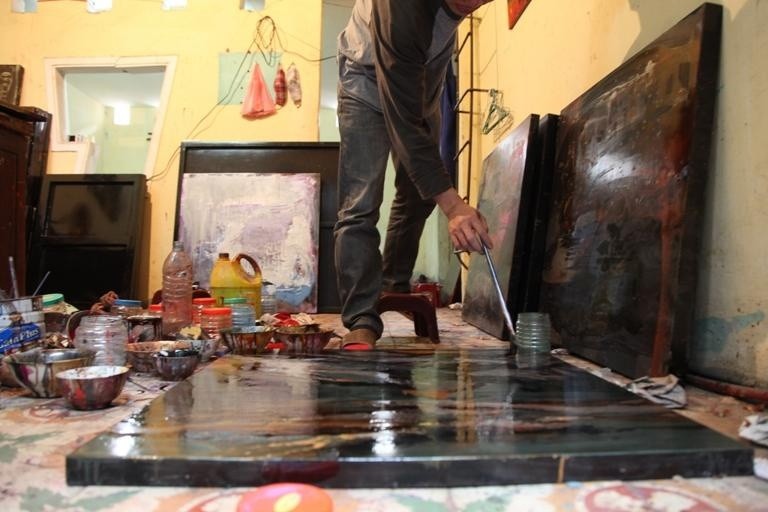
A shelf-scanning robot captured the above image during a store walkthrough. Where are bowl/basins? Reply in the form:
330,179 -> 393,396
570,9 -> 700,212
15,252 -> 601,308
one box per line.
54,366 -> 129,409
122,340 -> 219,381
221,326 -> 333,355
6,348 -> 95,397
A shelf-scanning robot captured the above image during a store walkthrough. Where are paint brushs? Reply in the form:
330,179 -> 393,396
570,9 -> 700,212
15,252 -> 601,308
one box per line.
483,242 -> 517,353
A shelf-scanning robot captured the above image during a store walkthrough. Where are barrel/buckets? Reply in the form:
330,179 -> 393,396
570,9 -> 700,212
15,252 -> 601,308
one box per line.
0,296 -> 46,356
211,251 -> 263,320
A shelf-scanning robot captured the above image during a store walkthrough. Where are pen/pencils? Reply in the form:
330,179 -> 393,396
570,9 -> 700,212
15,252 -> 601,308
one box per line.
32,270 -> 52,298
8,256 -> 19,298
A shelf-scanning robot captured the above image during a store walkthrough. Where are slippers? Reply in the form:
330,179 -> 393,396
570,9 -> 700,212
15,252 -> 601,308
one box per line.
342,328 -> 376,350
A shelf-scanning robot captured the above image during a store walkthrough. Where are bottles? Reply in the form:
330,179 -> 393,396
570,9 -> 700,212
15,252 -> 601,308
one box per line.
111,299 -> 162,316
516,312 -> 552,369
192,297 -> 256,354
73,315 -> 129,366
162,241 -> 192,337
41,294 -> 68,347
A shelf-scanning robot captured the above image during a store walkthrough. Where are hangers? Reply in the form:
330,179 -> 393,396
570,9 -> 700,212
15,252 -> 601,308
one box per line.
482,87 -> 508,135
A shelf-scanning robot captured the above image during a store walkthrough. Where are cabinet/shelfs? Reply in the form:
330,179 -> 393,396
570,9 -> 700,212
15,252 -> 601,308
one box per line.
0,109 -> 34,296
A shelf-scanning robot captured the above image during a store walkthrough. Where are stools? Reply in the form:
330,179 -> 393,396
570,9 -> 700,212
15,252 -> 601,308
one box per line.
379,292 -> 440,344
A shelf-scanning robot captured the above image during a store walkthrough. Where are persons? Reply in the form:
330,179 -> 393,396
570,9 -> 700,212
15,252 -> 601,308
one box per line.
334,1 -> 494,350
